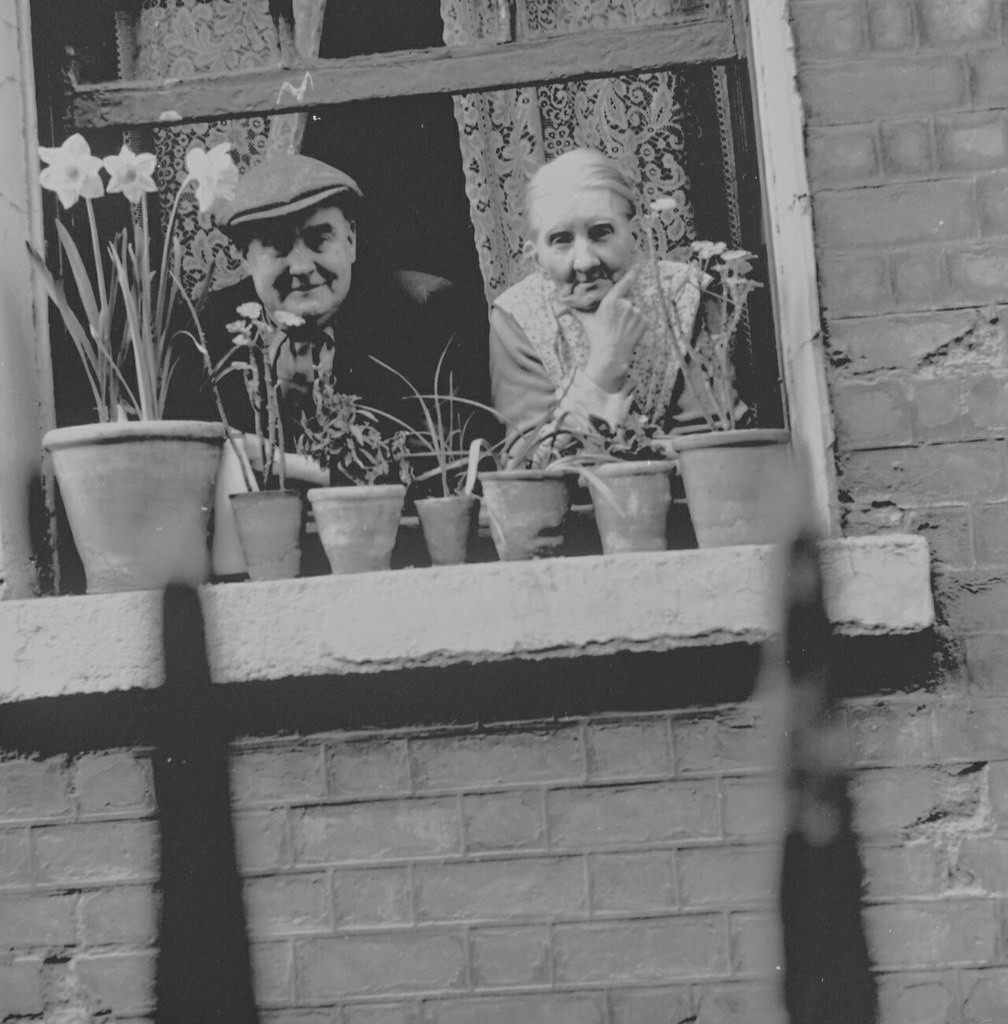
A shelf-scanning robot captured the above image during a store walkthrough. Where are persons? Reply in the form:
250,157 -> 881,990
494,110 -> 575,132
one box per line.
489,149 -> 754,472
213,154 -> 504,517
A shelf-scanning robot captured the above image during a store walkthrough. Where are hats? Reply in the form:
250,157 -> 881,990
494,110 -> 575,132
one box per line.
209,150 -> 365,228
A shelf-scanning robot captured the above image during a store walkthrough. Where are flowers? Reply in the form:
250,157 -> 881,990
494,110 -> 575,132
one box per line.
18,128 -> 766,492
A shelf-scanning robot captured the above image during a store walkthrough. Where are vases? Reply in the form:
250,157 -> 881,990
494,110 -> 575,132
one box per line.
47,420 -> 794,592
